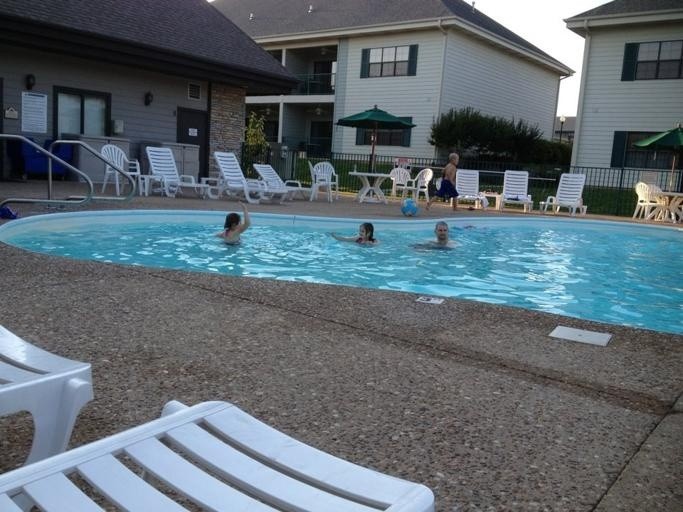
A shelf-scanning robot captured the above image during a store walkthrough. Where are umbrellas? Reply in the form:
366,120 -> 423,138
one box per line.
631,123 -> 683,192
336,105 -> 416,173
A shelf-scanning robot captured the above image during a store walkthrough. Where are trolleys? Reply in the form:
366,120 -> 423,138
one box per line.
6,134 -> 74,183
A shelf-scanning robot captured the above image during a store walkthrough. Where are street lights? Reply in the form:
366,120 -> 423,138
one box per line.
558,113 -> 566,144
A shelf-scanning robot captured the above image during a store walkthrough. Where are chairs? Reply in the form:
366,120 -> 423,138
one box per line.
630,180 -> 682,223
19,136 -> 76,182
0,316 -> 92,511
98,141 -> 342,203
346,165 -> 591,217
0,393 -> 441,512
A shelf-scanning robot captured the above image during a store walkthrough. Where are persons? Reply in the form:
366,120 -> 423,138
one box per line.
426,221 -> 460,249
215,200 -> 250,245
331,223 -> 376,244
426,152 -> 460,211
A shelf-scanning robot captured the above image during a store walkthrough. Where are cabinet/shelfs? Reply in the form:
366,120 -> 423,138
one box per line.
62,131 -> 132,185
